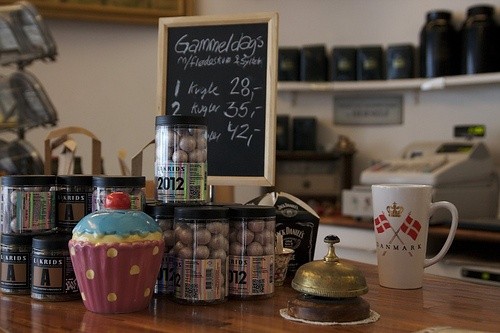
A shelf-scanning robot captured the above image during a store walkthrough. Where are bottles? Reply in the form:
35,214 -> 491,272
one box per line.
460,5 -> 499,74
420,12 -> 457,79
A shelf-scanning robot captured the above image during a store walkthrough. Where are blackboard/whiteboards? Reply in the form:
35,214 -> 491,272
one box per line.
156,12 -> 279,189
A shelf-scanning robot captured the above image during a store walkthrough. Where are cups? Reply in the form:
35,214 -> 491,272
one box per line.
371,184 -> 458,290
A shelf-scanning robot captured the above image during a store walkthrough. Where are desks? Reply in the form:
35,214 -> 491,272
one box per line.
0,259 -> 500,333
